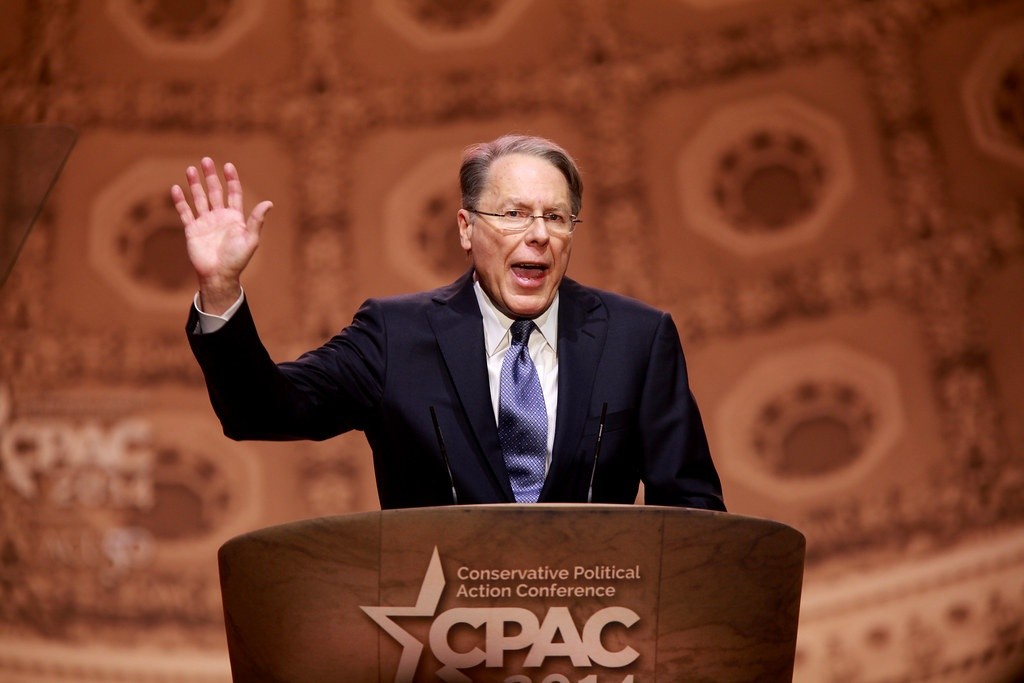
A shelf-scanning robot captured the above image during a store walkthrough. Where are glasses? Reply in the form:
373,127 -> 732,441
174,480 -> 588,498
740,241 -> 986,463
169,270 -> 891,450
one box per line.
465,208 -> 585,236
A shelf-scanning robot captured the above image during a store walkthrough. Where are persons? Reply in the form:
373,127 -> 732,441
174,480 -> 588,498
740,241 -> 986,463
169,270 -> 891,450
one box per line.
171,136 -> 726,511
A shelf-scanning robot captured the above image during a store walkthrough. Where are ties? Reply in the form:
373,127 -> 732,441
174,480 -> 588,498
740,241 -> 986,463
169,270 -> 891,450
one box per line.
498,317 -> 549,504
